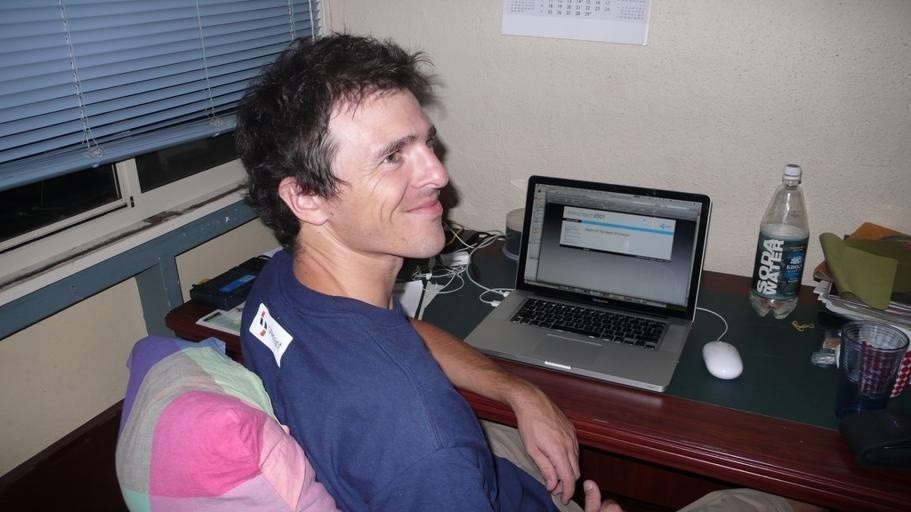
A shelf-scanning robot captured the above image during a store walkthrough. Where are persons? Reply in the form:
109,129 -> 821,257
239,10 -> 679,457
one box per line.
233,20 -> 823,511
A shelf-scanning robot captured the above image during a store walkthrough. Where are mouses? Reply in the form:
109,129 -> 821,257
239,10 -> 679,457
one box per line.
702,341 -> 744,379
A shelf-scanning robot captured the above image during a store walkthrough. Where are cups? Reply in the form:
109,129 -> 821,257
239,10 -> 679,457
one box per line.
835,321 -> 911,416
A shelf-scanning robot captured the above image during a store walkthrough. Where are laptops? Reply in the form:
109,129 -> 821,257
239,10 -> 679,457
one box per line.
463,175 -> 712,394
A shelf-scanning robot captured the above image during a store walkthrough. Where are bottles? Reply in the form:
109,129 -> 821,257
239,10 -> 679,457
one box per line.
502,209 -> 526,260
750,163 -> 810,320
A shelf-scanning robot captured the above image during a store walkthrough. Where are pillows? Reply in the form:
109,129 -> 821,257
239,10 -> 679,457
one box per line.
112,331 -> 334,511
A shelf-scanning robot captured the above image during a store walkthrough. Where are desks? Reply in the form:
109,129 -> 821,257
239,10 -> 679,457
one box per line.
162,238 -> 910,511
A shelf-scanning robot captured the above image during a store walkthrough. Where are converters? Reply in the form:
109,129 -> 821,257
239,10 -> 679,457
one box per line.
440,251 -> 471,266
456,231 -> 478,249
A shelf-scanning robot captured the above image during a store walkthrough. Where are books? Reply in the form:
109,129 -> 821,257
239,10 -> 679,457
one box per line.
813,259 -> 911,331
